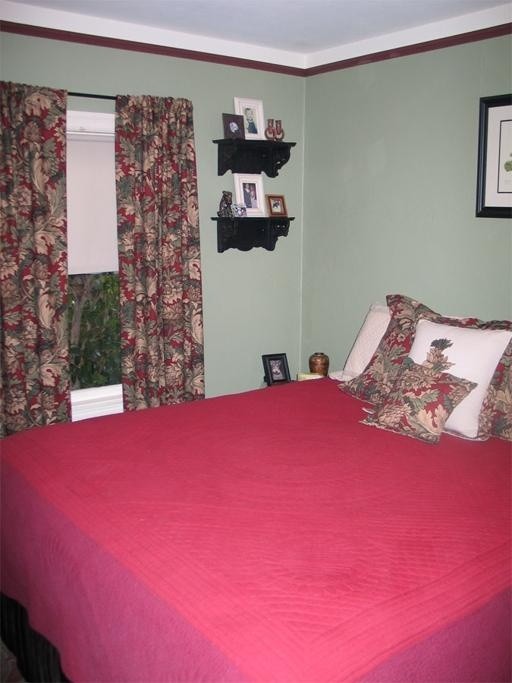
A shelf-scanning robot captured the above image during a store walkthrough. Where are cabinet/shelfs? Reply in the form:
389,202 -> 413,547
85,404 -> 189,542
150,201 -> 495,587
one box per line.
210,139 -> 297,253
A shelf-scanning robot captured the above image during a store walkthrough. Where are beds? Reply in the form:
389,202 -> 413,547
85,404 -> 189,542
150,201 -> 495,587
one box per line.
0,376 -> 512,683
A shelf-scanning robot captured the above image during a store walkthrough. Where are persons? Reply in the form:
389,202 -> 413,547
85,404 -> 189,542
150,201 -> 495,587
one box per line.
244,183 -> 258,208
245,108 -> 257,133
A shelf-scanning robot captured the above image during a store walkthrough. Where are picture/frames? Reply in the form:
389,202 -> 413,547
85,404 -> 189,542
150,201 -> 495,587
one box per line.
475,94 -> 512,218
234,172 -> 268,217
262,353 -> 291,386
222,113 -> 245,139
265,194 -> 288,217
234,96 -> 268,140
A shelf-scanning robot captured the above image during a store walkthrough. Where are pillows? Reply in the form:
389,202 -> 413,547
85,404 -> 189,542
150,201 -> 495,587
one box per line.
327,304 -> 476,382
336,294 -> 512,441
408,314 -> 512,441
358,354 -> 478,445
